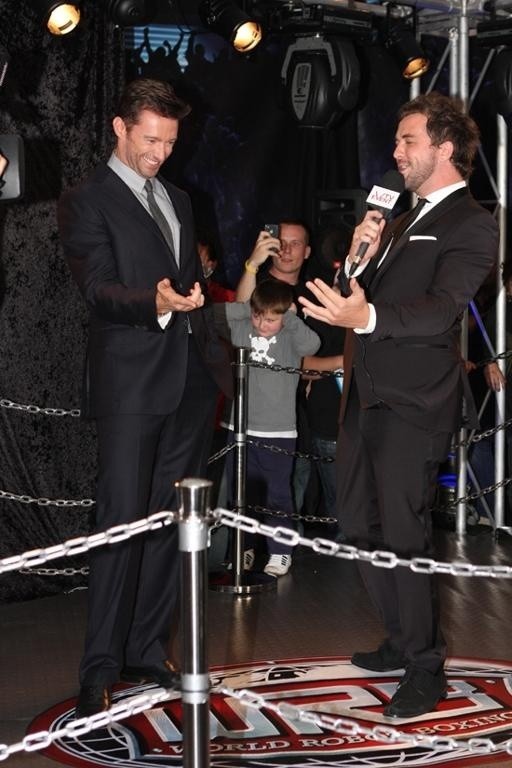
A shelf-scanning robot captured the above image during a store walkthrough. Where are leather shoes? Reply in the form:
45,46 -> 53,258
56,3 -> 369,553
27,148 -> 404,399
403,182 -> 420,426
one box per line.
120,656 -> 182,690
75,683 -> 112,720
384,672 -> 448,718
350,639 -> 409,671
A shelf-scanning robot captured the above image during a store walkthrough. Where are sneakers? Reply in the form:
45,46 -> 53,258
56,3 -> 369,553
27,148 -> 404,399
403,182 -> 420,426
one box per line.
263,553 -> 291,576
228,548 -> 256,569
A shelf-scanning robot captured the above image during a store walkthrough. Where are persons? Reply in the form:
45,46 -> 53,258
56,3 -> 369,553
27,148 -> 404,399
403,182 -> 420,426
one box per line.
212,279 -> 322,578
197,239 -> 236,435
465,360 -> 478,374
127,26 -> 229,93
57,77 -> 237,721
297,92 -> 502,720
482,275 -> 511,393
237,216 -> 346,544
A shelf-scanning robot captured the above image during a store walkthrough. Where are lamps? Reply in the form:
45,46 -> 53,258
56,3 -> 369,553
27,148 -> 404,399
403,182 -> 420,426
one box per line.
198,0 -> 430,79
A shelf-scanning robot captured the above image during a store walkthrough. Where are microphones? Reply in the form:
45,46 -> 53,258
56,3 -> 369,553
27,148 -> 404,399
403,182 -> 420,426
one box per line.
349,169 -> 404,278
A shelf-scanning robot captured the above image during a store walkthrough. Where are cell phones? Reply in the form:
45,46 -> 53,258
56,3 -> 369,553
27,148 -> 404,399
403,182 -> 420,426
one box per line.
264,224 -> 280,253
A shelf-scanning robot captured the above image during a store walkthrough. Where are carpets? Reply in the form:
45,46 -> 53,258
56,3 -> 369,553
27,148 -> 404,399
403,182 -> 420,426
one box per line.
26,650 -> 509,768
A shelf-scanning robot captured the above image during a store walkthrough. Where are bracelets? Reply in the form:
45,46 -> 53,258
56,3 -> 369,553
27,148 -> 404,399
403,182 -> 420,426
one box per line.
244,258 -> 260,275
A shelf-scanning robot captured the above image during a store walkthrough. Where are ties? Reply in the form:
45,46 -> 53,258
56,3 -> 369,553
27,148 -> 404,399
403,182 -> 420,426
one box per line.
145,180 -> 174,257
385,198 -> 428,257
